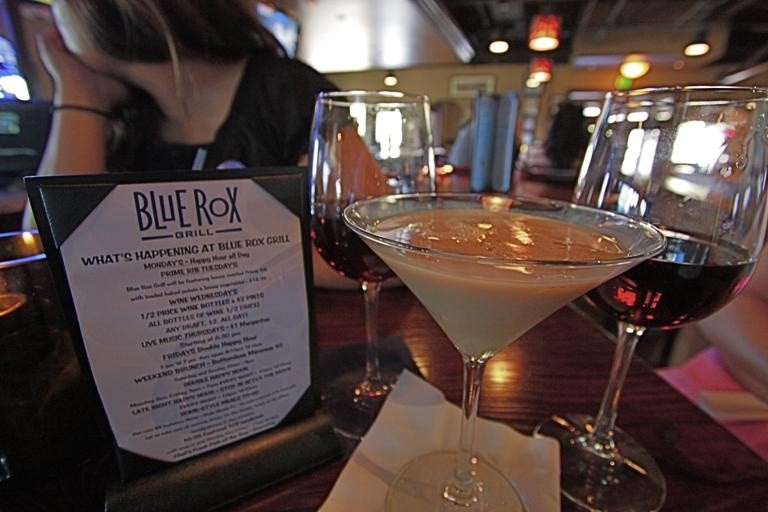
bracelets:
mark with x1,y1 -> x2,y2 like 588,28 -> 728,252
47,103 -> 110,120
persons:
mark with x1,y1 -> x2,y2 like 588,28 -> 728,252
22,2 -> 404,290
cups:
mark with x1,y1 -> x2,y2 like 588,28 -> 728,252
0,230 -> 72,426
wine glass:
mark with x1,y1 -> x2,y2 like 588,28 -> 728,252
345,193 -> 669,512
533,83 -> 768,512
308,90 -> 438,441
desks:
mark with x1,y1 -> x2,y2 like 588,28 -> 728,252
0,291 -> 768,511
